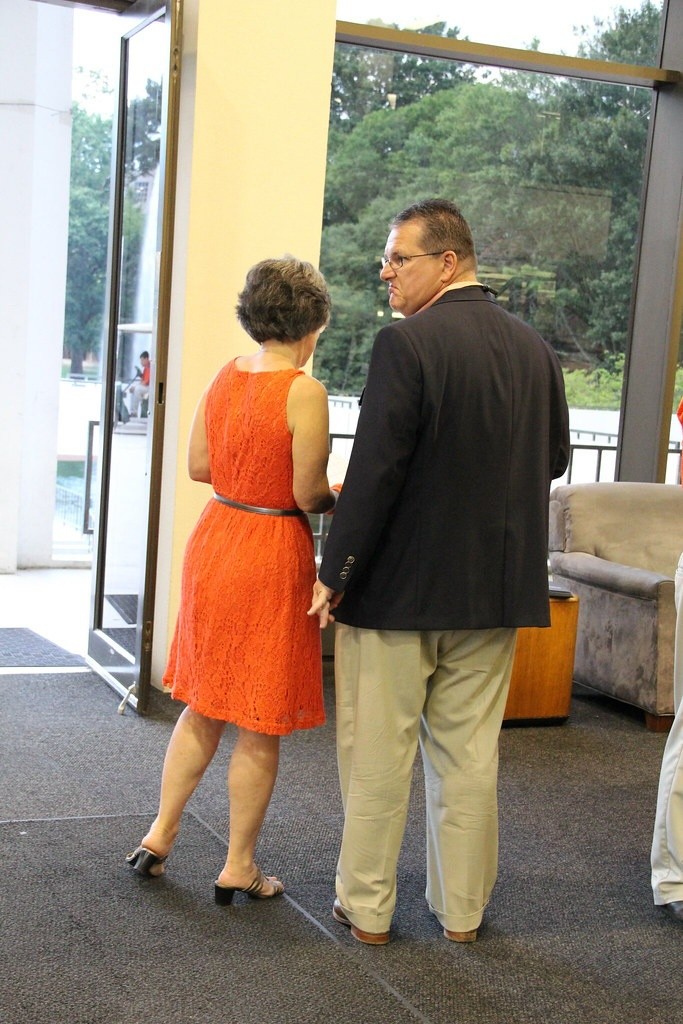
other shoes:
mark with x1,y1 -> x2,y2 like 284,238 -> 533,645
657,901 -> 683,922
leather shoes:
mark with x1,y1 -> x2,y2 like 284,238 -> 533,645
332,897 -> 390,944
442,920 -> 477,941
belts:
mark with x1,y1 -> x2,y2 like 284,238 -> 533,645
214,493 -> 303,517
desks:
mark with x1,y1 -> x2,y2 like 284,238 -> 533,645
501,593 -> 579,729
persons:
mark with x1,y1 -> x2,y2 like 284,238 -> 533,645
124,256 -> 343,908
305,199 -> 571,946
128,351 -> 151,417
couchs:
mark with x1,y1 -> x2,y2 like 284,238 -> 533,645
548,481 -> 683,732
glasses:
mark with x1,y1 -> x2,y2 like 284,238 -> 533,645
381,249 -> 457,270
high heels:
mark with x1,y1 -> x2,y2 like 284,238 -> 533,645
125,846 -> 166,877
213,870 -> 284,905
